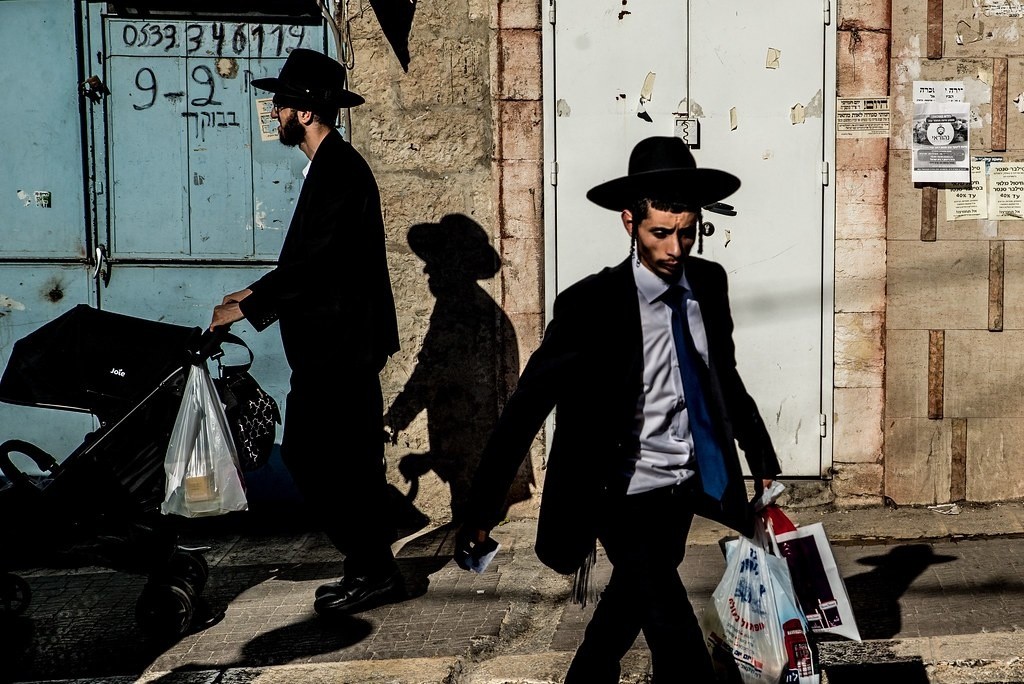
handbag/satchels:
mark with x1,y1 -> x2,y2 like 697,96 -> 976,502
212,340 -> 280,474
161,363 -> 249,519
700,481 -> 862,684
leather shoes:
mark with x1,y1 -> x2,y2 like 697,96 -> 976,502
314,566 -> 405,617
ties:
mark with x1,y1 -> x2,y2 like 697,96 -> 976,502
660,285 -> 730,502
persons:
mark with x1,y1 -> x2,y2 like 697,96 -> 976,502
210,48 -> 405,618
451,137 -> 781,684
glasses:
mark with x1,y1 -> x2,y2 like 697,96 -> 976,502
271,96 -> 299,112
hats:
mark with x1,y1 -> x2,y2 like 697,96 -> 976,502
587,136 -> 741,212
250,49 -> 367,109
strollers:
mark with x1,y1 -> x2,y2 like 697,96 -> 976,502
0,300 -> 234,645
